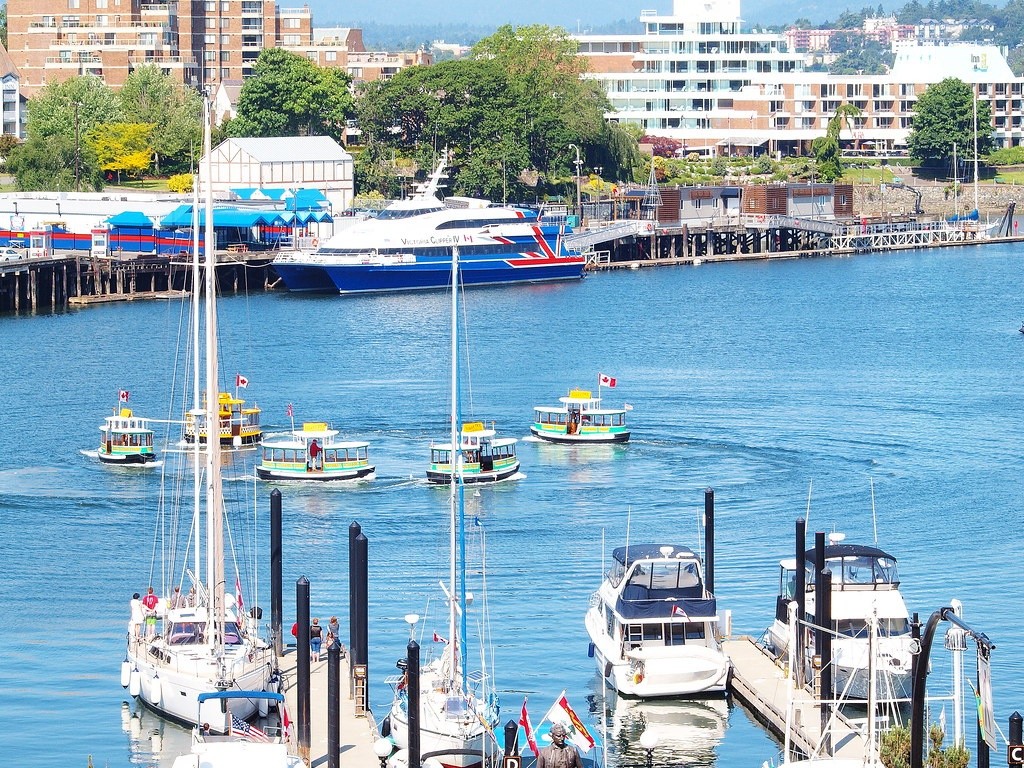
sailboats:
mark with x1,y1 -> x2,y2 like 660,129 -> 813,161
382,247 -> 502,768
123,47 -> 275,737
946,86 -> 1000,233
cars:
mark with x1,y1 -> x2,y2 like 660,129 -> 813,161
340,208 -> 383,218
0,249 -> 23,262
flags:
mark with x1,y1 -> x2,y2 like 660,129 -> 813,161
236,374 -> 250,389
433,631 -> 449,645
598,372 -> 617,388
231,714 -> 270,743
118,389 -> 129,403
518,689 -> 597,756
235,575 -> 244,628
286,403 -> 294,417
280,700 -> 291,740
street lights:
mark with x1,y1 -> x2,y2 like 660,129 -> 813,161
68,102 -> 83,193
808,160 -> 817,220
569,144 -> 582,206
862,160 -> 869,212
594,167 -> 603,230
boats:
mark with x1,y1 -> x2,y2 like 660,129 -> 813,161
766,476 -> 932,712
309,115 -> 585,295
270,166 -> 368,292
584,499 -> 733,699
253,423 -> 375,481
165,489 -> 518,768
183,392 -> 261,445
529,387 -> 631,443
97,407 -> 156,464
426,419 -> 520,485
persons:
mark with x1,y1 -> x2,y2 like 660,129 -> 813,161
465,436 -> 476,463
292,615 -> 339,663
535,722 -> 586,768
309,439 -> 323,471
221,405 -> 232,427
204,723 -> 211,736
130,586 -> 159,643
170,586 -> 197,610
325,454 -> 335,462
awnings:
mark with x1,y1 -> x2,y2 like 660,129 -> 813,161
229,188 -> 332,211
714,137 -> 770,147
